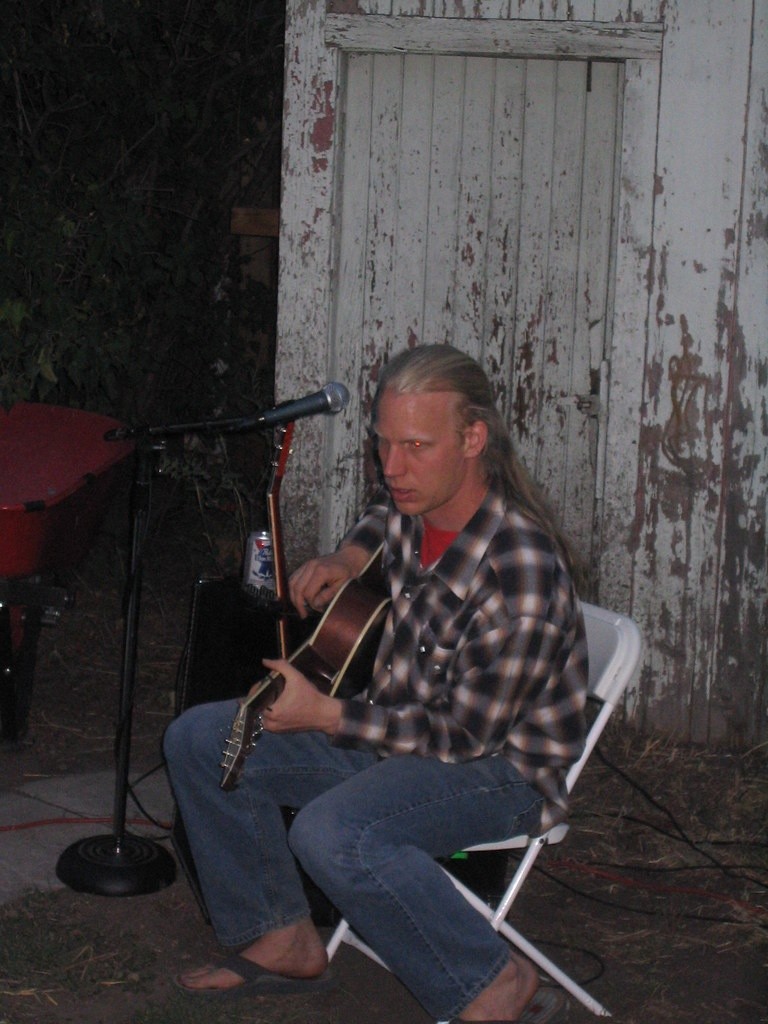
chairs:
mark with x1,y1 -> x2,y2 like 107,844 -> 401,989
327,599 -> 643,1024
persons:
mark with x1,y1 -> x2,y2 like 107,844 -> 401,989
163,345 -> 588,1024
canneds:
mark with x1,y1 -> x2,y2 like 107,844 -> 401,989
243,531 -> 273,585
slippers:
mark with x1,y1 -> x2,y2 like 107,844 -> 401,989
438,986 -> 572,1024
171,953 -> 339,1000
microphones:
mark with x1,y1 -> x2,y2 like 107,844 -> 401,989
242,381 -> 350,428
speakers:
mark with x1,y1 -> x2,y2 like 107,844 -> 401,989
178,580 -> 325,715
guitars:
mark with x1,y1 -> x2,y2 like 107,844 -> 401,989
216,536 -> 393,795
